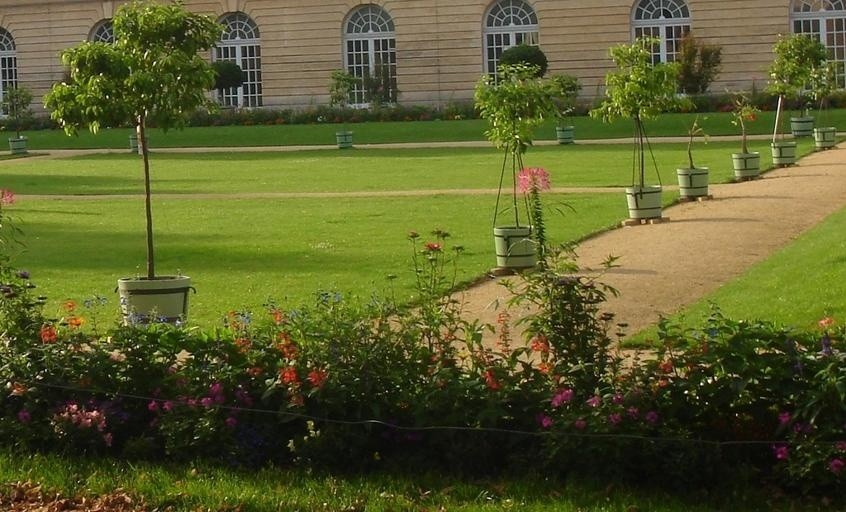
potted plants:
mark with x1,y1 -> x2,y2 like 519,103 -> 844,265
42,0 -> 231,326
328,69 -> 364,148
474,61 -> 583,267
0,82 -> 35,154
